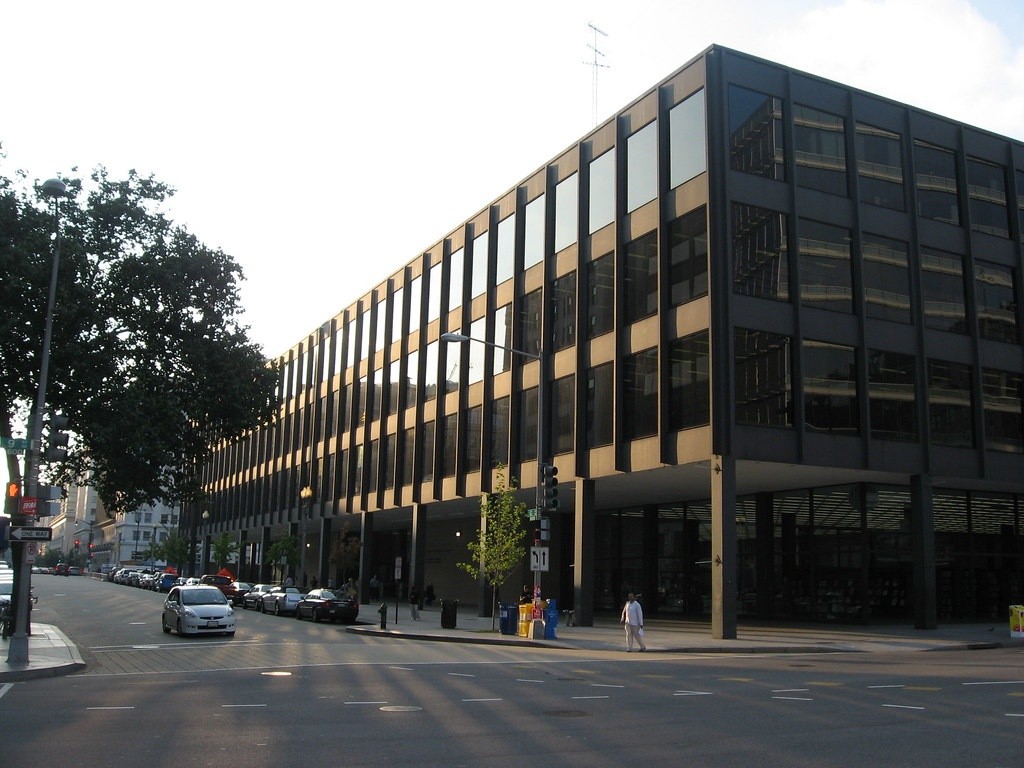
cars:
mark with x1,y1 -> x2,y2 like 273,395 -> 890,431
0,560 -> 82,604
161,585 -> 235,638
107,566 -> 201,592
295,588 -> 360,624
242,584 -> 278,612
224,581 -> 256,607
261,584 -> 310,616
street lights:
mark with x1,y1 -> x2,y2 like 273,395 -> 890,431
439,332 -> 551,621
301,486 -> 313,586
150,522 -> 159,572
200,510 -> 209,576
134,518 -> 141,564
74,519 -> 94,565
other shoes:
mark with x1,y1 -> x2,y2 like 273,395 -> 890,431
627,648 -> 632,651
638,646 -> 646,652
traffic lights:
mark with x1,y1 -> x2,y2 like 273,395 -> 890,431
89,544 -> 93,549
543,465 -> 558,509
6,482 -> 21,500
39,484 -> 63,517
75,540 -> 78,549
46,413 -> 69,462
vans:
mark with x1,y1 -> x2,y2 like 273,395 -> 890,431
194,575 -> 231,594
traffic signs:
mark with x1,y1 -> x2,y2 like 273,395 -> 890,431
8,526 -> 53,541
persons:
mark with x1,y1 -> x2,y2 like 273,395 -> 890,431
370,575 -> 380,601
144,569 -> 149,573
346,578 -> 356,591
310,576 -> 319,590
409,586 -> 420,620
620,591 -> 646,652
520,585 -> 534,604
426,582 -> 434,606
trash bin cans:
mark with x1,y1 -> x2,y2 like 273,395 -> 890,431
498,602 -> 518,635
439,598 -> 460,629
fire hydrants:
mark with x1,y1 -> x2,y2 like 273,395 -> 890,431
378,603 -> 388,629
